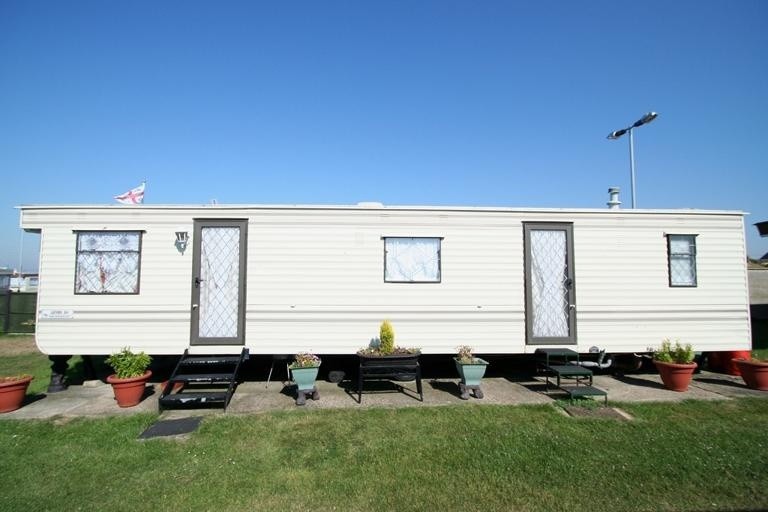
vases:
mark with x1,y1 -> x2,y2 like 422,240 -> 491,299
729,354 -> 767,392
286,364 -> 321,390
450,356 -> 490,386
0,375 -> 36,412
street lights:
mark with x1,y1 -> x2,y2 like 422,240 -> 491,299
604,109 -> 659,211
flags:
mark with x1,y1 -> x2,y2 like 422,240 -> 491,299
114,183 -> 144,204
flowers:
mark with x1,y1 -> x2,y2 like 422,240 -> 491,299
453,345 -> 474,364
291,351 -> 317,367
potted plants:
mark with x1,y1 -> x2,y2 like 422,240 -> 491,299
357,320 -> 421,372
645,338 -> 697,392
102,345 -> 155,407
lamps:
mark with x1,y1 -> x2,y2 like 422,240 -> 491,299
173,226 -> 188,249
